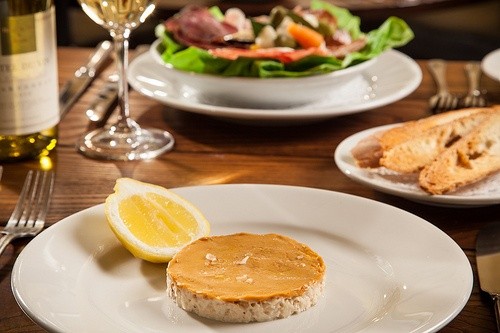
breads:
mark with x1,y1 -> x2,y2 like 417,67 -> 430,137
166,232 -> 327,323
351,105 -> 500,195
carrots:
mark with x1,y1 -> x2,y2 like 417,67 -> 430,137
288,23 -> 323,49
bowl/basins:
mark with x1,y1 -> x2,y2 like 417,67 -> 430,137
146,28 -> 381,107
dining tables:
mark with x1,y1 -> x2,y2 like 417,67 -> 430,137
0,46 -> 500,333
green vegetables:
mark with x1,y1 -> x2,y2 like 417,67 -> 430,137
159,0 -> 414,78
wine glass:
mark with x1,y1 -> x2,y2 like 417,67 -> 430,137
75,0 -> 177,161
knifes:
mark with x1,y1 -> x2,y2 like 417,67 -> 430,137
472,223 -> 500,332
60,41 -> 113,119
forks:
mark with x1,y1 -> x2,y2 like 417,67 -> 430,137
461,62 -> 487,107
0,169 -> 54,256
427,59 -> 458,110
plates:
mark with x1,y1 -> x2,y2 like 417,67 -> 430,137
333,123 -> 500,207
128,44 -> 422,119
482,48 -> 500,86
11,184 -> 473,332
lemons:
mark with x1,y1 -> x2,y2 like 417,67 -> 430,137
104,178 -> 211,263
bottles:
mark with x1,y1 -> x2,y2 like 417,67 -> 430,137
0,1 -> 60,165
